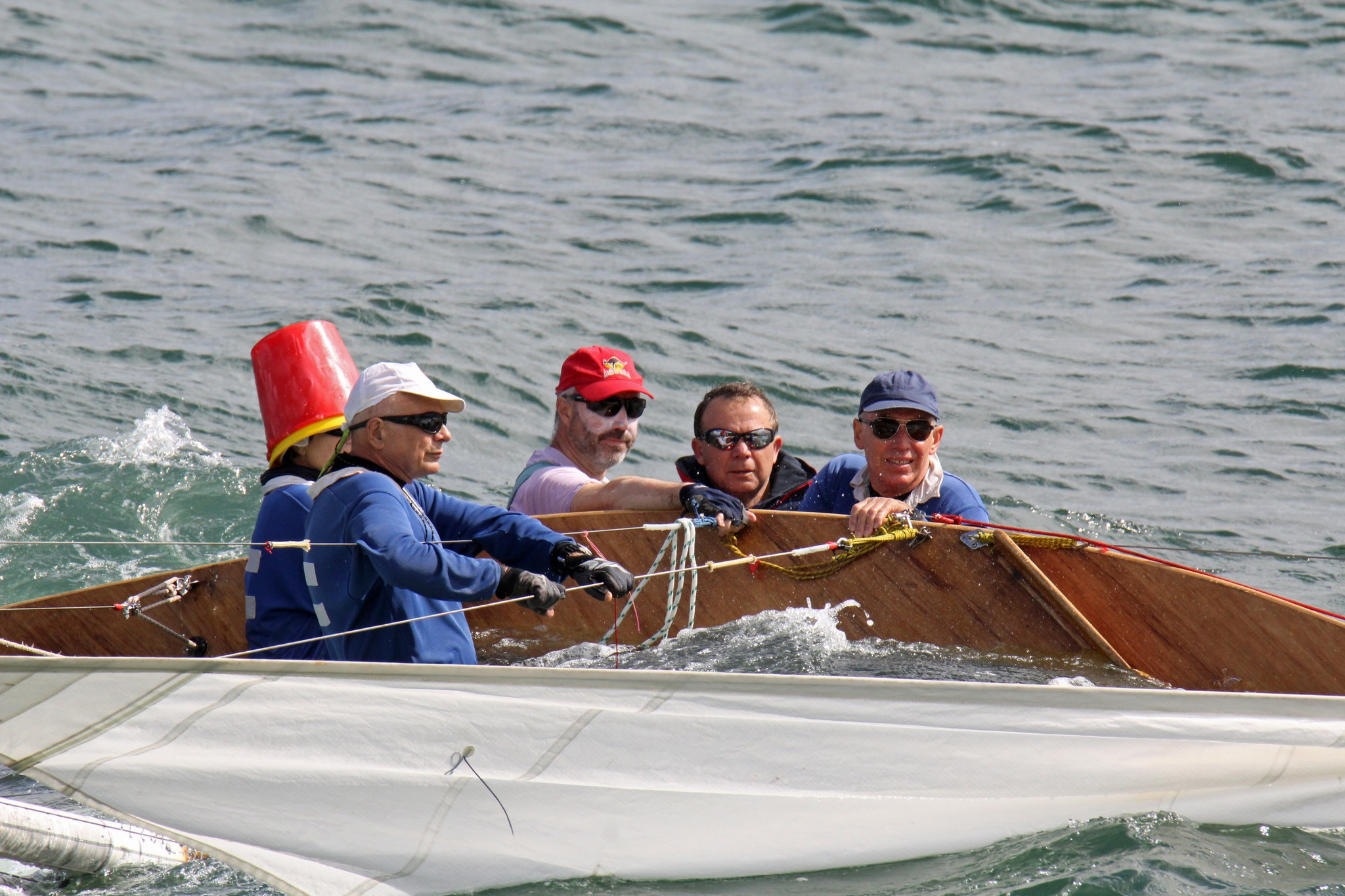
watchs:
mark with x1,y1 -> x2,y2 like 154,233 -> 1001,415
556,543 -> 592,569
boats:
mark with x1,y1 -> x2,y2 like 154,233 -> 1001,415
0,504 -> 1343,896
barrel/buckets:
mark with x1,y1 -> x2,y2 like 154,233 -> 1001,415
251,319 -> 361,468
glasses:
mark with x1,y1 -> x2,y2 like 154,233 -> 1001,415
378,412 -> 448,433
327,428 -> 351,437
698,429 -> 775,450
562,394 -> 646,418
858,417 -> 937,441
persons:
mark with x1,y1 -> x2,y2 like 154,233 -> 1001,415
302,362 -> 634,664
674,382 -> 817,513
798,371 -> 990,539
245,320 -> 362,661
506,345 -> 756,536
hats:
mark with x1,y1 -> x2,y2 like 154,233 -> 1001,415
556,345 -> 654,401
858,370 -> 940,420
344,362 -> 466,425
